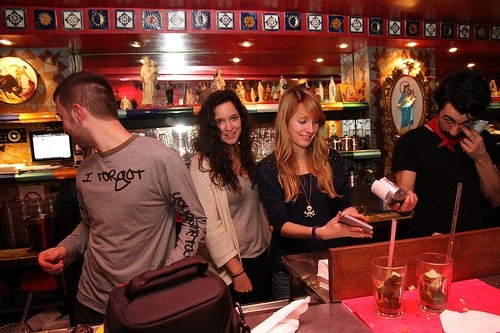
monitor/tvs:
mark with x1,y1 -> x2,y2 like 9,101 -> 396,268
28,130 -> 73,166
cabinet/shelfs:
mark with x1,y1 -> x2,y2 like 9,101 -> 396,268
0,98 -> 500,265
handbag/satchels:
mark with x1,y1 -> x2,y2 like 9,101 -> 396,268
104,255 -> 251,333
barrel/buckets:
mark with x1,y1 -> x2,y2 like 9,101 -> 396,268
21,213 -> 58,250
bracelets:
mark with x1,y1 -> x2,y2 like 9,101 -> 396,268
233,270 -> 245,278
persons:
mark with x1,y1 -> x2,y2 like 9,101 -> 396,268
38,72 -> 207,328
189,90 -> 272,305
257,87 -> 373,300
235,81 -> 246,101
397,85 -> 416,127
388,72 -> 500,236
140,56 -> 159,104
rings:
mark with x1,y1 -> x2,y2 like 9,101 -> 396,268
338,211 -> 344,221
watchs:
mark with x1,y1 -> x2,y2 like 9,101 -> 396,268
312,226 -> 320,240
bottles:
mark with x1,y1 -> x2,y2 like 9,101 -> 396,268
366,169 -> 377,185
74,145 -> 84,166
347,167 -> 358,188
325,131 -> 370,151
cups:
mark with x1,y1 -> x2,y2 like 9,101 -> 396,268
415,253 -> 454,315
371,256 -> 408,319
144,126 -> 198,165
0,323 -> 31,333
0,192 -> 61,248
248,126 -> 275,158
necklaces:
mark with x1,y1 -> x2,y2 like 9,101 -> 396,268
299,172 -> 315,217
